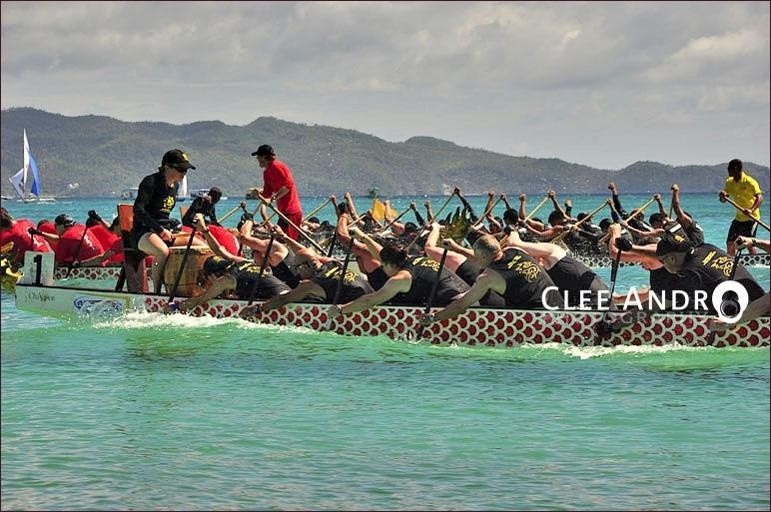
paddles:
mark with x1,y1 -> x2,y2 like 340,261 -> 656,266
319,215 -> 366,247
551,200 -> 608,242
499,196 -> 550,250
597,198 -> 655,246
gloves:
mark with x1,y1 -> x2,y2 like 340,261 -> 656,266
27,226 -> 43,236
613,237 -> 633,253
86,210 -> 103,221
592,321 -> 612,338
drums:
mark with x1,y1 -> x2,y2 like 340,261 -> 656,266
162,244 -> 212,295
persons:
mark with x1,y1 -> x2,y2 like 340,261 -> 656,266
0,145 -> 771,327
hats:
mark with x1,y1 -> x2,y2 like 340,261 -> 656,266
204,255 -> 235,272
250,146 -> 277,158
55,214 -> 75,227
162,148 -> 196,173
656,233 -> 691,257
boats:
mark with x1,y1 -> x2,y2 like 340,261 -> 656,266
729,252 -> 771,271
565,253 -> 645,272
11,276 -> 770,350
0,267 -> 131,287
